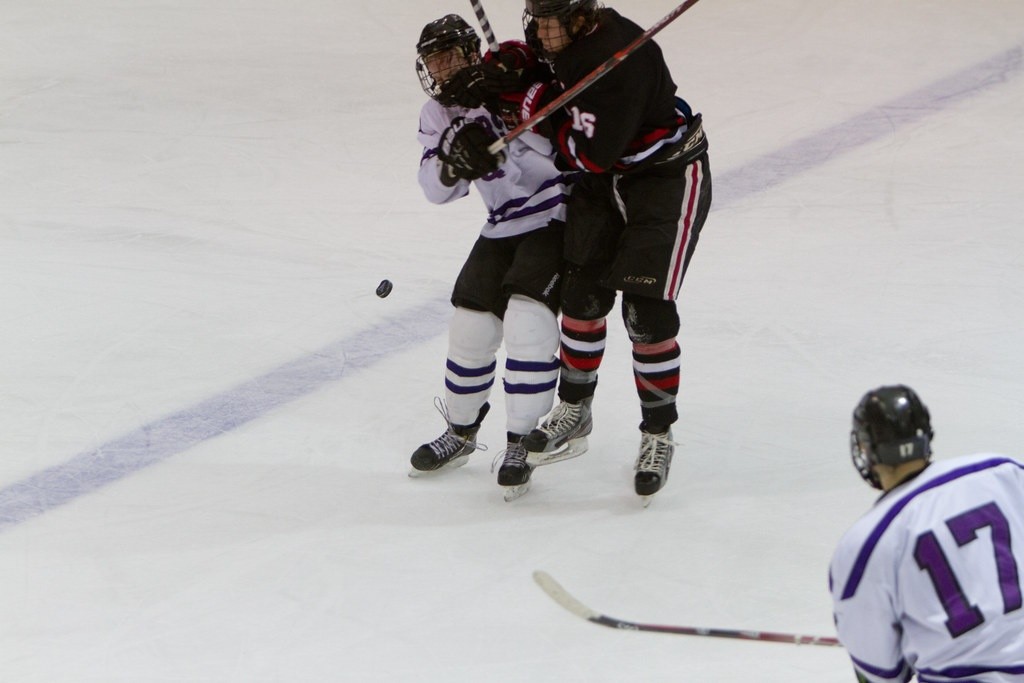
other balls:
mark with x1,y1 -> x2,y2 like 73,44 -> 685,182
375,278 -> 395,299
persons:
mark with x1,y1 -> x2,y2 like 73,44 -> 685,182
829,385 -> 1024,683
408,0 -> 712,508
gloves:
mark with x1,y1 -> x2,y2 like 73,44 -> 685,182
437,116 -> 506,177
497,82 -> 548,133
482,39 -> 535,93
440,64 -> 486,108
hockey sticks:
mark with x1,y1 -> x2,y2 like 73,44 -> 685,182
486,0 -> 701,159
529,565 -> 848,650
466,0 -> 505,61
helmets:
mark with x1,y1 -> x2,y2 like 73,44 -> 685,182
851,383 -> 934,490
416,14 -> 481,104
525,0 -> 599,61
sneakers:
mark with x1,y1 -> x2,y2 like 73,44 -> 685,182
491,431 -> 537,502
522,373 -> 598,466
408,396 -> 491,478
633,421 -> 685,508
